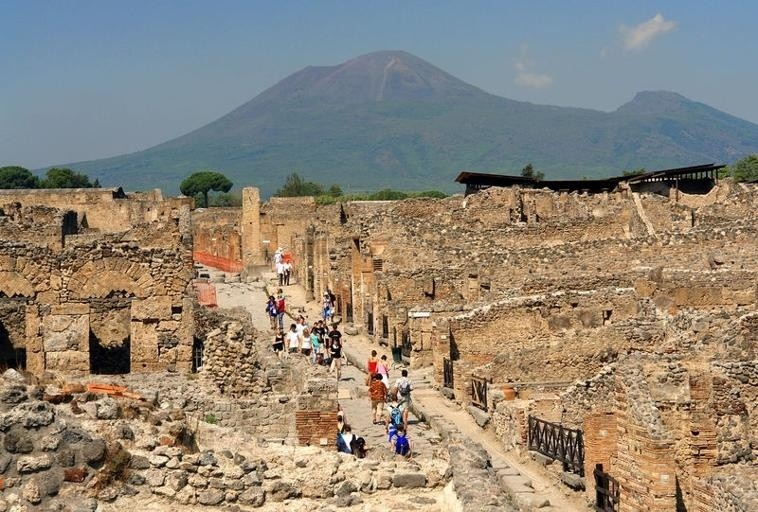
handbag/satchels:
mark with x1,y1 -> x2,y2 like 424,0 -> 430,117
349,434 -> 356,455
398,388 -> 408,396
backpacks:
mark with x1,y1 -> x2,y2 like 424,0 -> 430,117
394,433 -> 409,456
389,404 -> 402,425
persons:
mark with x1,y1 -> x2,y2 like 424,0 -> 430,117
263,246 -> 417,459
